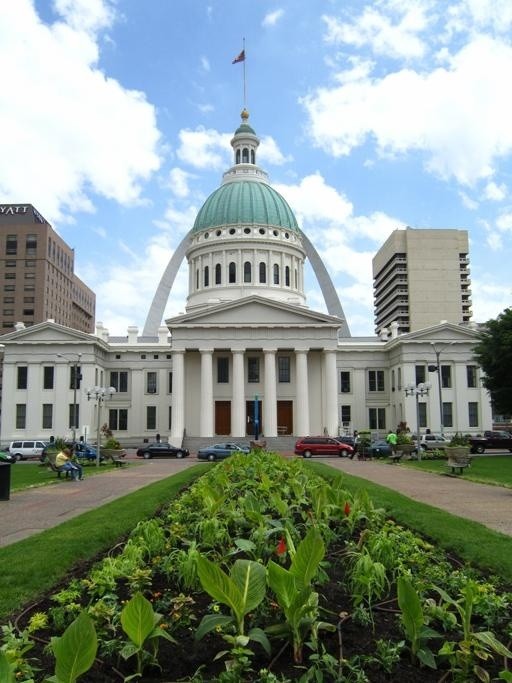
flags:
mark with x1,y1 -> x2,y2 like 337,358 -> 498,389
231,48 -> 245,64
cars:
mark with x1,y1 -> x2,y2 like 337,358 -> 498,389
333,436 -> 363,454
137,442 -> 190,459
364,438 -> 396,458
41,442 -> 104,465
198,442 -> 251,461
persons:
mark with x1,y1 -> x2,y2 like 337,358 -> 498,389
350,431 -> 361,459
56,448 -> 79,481
69,449 -> 85,480
387,430 -> 397,453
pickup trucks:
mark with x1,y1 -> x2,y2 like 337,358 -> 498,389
468,431 -> 512,454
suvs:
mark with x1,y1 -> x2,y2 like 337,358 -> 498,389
419,434 -> 452,452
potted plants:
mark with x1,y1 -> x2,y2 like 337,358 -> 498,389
100,439 -> 124,463
48,439 -> 69,465
444,432 -> 472,463
397,432 -> 417,459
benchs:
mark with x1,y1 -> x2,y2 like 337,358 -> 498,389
111,455 -> 126,467
445,455 -> 472,474
388,450 -> 404,463
49,460 -> 69,478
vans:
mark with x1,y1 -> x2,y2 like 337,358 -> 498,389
295,436 -> 357,459
8,440 -> 48,461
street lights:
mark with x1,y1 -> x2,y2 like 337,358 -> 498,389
429,339 -> 456,444
86,385 -> 116,466
56,351 -> 82,443
